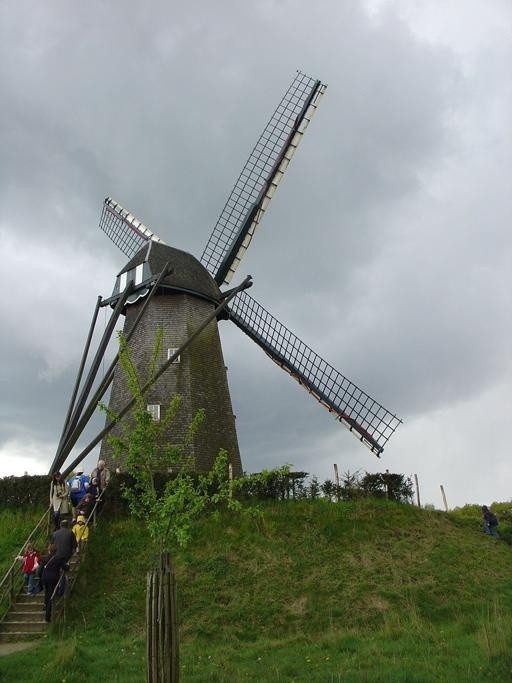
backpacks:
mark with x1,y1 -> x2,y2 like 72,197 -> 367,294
70,475 -> 84,492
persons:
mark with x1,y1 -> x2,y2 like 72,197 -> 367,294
65,471 -> 89,521
49,518 -> 78,565
480,503 -> 499,535
89,458 -> 111,510
73,476 -> 100,522
15,544 -> 41,594
37,541 -> 71,622
72,515 -> 89,543
90,476 -> 101,498
47,471 -> 69,519
